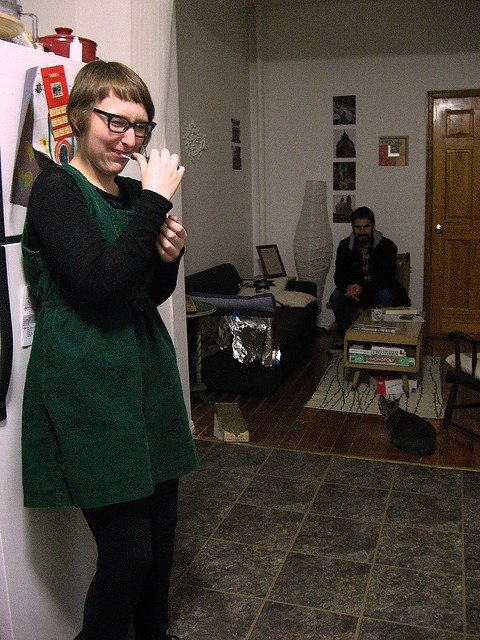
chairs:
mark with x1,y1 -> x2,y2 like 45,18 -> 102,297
326,252 -> 411,326
441,331 -> 480,441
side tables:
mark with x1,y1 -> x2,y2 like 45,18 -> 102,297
186,297 -> 217,407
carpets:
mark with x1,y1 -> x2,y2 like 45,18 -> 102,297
304,350 -> 446,419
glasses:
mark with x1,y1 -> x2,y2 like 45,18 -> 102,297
94,108 -> 157,138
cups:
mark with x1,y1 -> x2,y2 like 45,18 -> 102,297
371,305 -> 383,324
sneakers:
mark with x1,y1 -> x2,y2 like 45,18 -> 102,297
328,339 -> 344,353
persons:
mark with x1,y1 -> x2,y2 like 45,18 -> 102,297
22,62 -> 202,640
325,205 -> 412,356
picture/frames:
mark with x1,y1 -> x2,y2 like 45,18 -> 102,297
256,245 -> 286,278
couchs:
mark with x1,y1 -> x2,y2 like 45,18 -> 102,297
185,263 -> 318,397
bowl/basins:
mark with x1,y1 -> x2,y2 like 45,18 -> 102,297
0,12 -> 24,40
